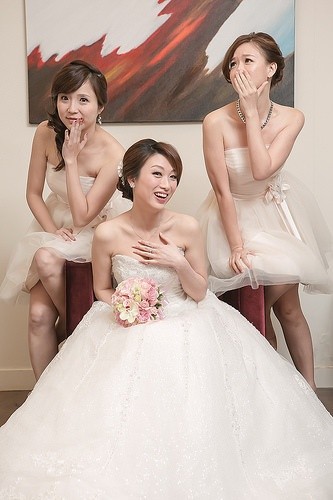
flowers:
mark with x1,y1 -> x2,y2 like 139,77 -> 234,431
109,273 -> 171,329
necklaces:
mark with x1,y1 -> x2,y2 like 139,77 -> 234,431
131,210 -> 166,241
235,100 -> 273,132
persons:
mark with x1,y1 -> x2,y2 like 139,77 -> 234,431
0,61 -> 125,382
203,32 -> 333,393
0,139 -> 333,500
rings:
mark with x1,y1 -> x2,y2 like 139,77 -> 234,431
148,248 -> 153,252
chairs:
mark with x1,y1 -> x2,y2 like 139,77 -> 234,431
65,259 -> 266,338
